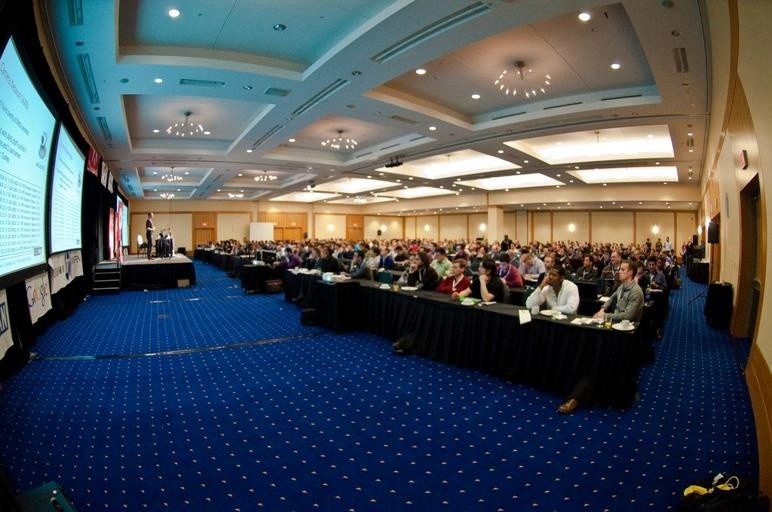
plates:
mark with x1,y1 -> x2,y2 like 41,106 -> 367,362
613,321 -> 635,332
380,286 -> 391,289
461,300 -> 475,306
401,285 -> 419,292
540,309 -> 562,316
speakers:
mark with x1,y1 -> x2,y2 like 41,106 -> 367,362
703,281 -> 733,330
178,248 -> 186,256
708,222 -> 719,244
693,235 -> 698,246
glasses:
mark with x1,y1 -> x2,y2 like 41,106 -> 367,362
544,261 -> 553,263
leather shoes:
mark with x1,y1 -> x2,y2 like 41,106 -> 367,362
558,398 -> 580,413
393,341 -> 404,353
657,328 -> 662,340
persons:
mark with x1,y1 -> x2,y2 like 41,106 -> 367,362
416,236 -> 545,302
682,237 -> 693,256
201,239 -> 421,284
392,252 -> 438,352
558,261 -> 644,411
525,236 -> 679,338
146,211 -> 155,260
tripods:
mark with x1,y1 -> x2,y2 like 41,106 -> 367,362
688,245 -> 721,305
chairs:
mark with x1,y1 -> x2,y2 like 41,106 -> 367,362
195,238 -> 678,412
136,234 -> 148,258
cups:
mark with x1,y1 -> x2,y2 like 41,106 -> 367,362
603,313 -> 613,330
464,297 -> 472,303
621,320 -> 630,329
531,305 -> 540,315
393,281 -> 400,292
382,284 -> 388,288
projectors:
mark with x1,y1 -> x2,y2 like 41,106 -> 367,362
385,162 -> 402,168
